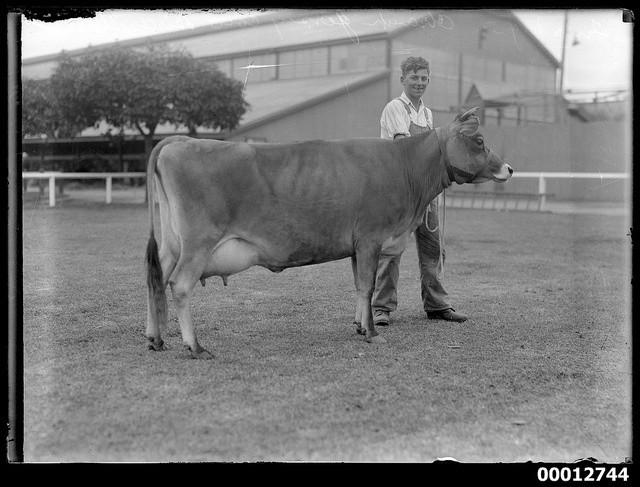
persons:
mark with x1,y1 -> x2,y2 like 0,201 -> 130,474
371,55 -> 469,324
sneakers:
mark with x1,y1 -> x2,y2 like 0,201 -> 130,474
427,308 -> 469,322
373,310 -> 390,325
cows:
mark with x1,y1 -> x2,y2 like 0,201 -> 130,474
143,106 -> 514,360
